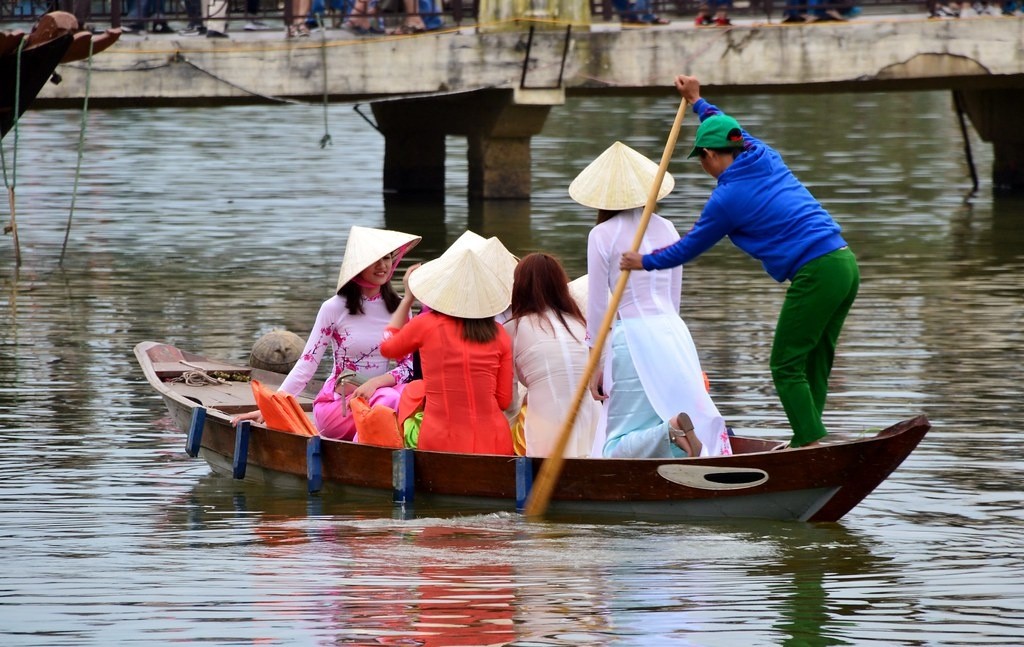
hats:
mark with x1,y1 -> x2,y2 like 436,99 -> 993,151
686,114 -> 744,160
567,273 -> 619,324
567,140 -> 675,210
441,230 -> 521,264
408,249 -> 512,320
473,237 -> 519,297
335,225 -> 422,294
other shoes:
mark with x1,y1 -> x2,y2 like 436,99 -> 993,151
346,20 -> 383,34
621,18 -> 654,29
206,30 -> 227,37
121,25 -> 145,35
245,23 -> 269,29
651,17 -> 670,25
178,26 -> 202,36
288,23 -> 309,38
390,25 -> 424,34
151,22 -> 175,34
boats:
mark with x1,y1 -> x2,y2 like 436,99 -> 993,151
0,11 -> 125,145
134,341 -> 933,525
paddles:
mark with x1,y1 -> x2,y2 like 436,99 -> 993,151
524,96 -> 689,518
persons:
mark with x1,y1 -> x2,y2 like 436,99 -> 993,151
695,0 -> 732,26
290,0 -> 443,38
568,141 -> 732,458
231,226 -> 421,441
613,0 -> 670,25
224,0 -> 268,30
179,0 -> 203,36
119,0 -> 175,35
379,249 -> 514,455
503,254 -> 603,458
443,232 -> 527,429
620,77 -> 860,448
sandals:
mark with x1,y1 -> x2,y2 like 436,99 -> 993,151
668,412 -> 702,457
